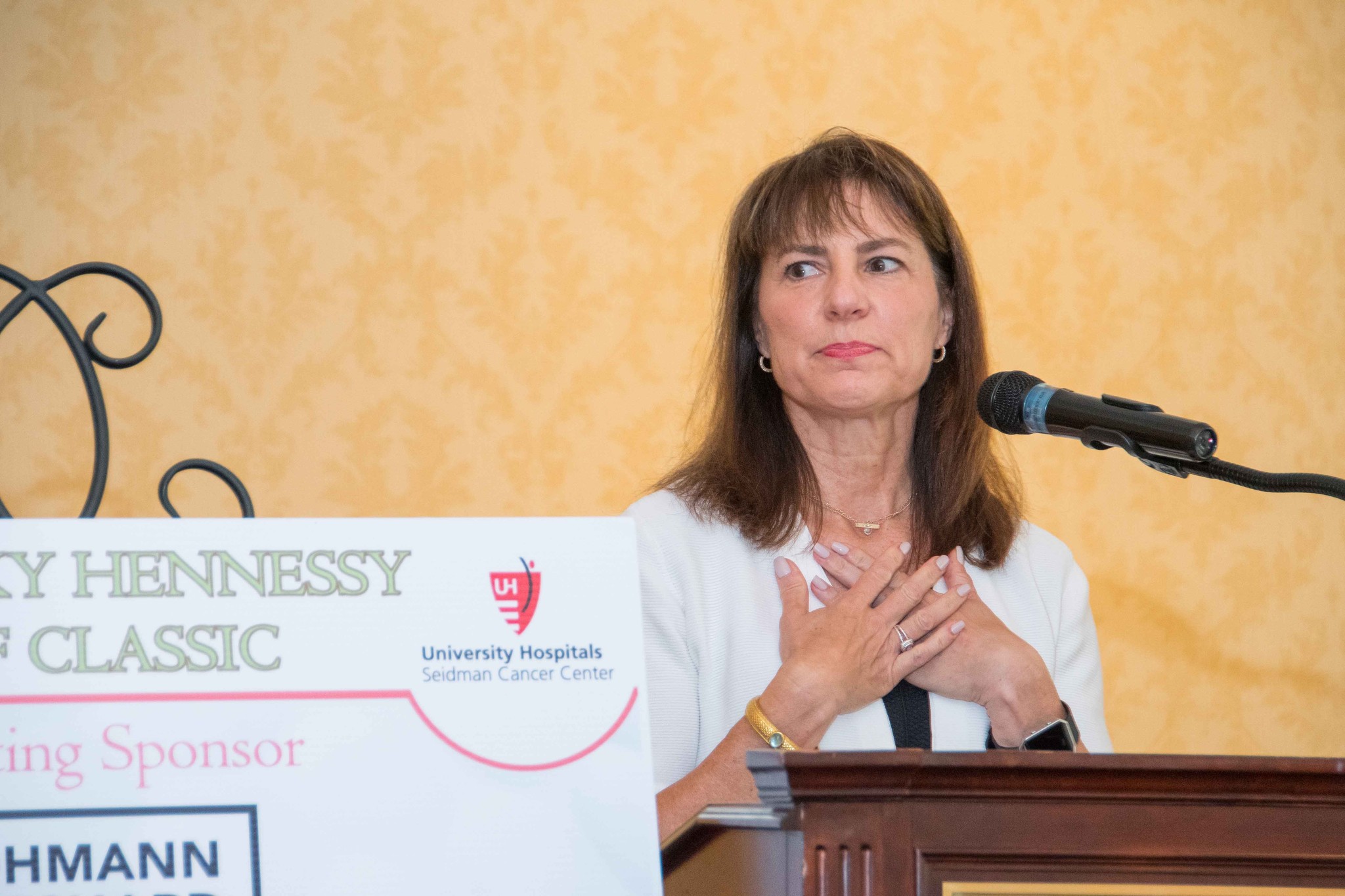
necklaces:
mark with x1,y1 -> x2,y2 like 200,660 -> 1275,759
801,490 -> 915,537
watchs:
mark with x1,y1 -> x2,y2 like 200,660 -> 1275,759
985,700 -> 1080,752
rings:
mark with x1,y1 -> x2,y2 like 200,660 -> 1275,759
893,625 -> 914,655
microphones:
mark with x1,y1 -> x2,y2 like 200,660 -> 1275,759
976,370 -> 1218,463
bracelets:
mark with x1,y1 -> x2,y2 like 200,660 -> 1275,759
744,695 -> 802,751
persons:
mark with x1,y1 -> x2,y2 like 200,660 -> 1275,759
629,132 -> 1116,853
